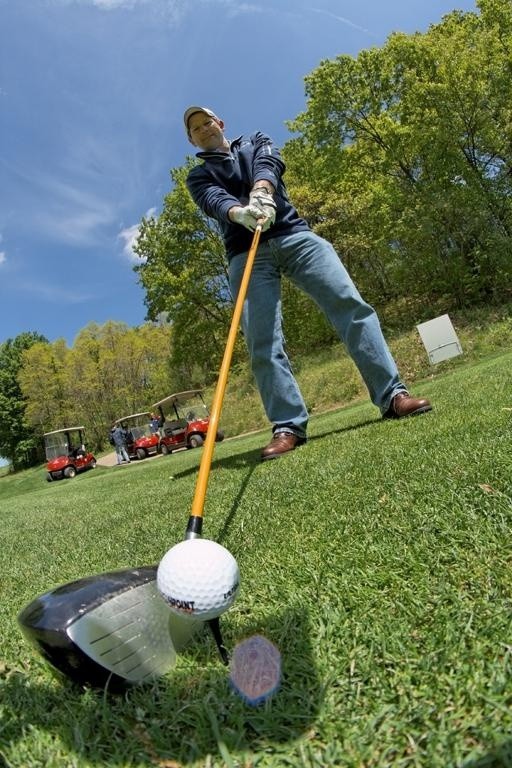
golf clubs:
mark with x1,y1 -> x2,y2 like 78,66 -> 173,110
18,219 -> 261,698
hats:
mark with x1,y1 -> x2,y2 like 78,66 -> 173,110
183,106 -> 220,128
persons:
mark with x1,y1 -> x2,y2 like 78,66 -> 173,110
150,413 -> 162,440
109,422 -> 130,465
183,106 -> 432,460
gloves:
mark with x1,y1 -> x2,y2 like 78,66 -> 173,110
233,186 -> 278,233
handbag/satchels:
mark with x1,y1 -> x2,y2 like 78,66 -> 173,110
110,438 -> 115,445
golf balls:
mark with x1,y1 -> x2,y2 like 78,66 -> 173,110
158,536 -> 237,621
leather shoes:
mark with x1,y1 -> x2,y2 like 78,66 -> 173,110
260,427 -> 307,461
381,391 -> 433,419
